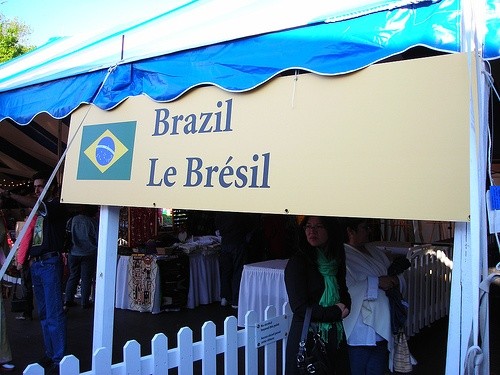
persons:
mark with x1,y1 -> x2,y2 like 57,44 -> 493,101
341,218 -> 399,375
62,205 -> 97,309
0,170 -> 67,375
284,216 -> 351,375
15,212 -> 36,321
218,210 -> 295,310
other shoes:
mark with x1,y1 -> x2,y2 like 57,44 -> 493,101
221,298 -> 228,305
0,362 -> 15,372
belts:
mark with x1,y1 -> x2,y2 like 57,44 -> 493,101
33,252 -> 59,262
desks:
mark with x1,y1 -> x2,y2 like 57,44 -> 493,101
238,259 -> 291,327
115,254 -> 224,314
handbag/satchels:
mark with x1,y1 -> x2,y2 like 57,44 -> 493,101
296,306 -> 330,375
63,217 -> 73,249
10,267 -> 34,312
392,331 -> 413,373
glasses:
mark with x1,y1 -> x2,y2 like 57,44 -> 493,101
303,225 -> 326,230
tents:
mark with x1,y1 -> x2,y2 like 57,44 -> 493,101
0,0 -> 500,375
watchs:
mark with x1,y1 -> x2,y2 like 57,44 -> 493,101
5,189 -> 11,198
